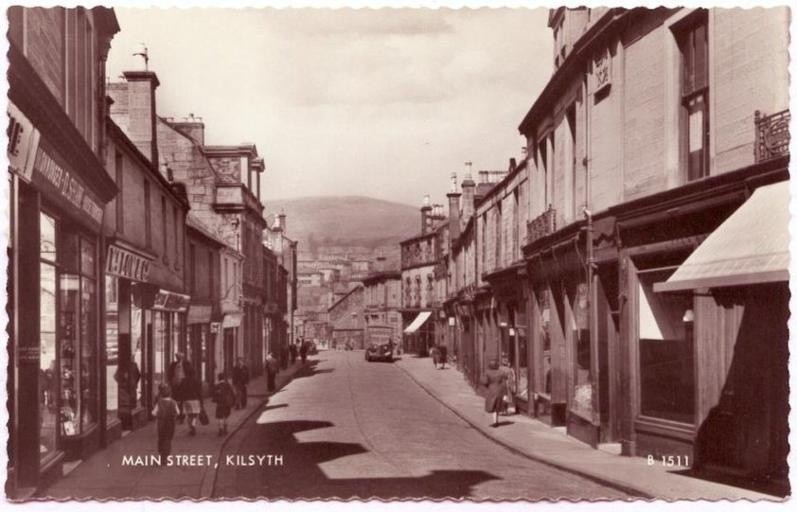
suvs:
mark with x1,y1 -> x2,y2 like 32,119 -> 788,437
364,334 -> 394,362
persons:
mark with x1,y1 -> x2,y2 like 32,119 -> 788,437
479,360 -> 508,427
150,385 -> 180,467
167,353 -> 195,400
177,365 -> 202,434
231,357 -> 249,409
299,344 -> 306,364
279,342 -> 288,370
38,360 -> 54,436
265,352 -> 279,392
393,335 -> 400,358
289,341 -> 297,364
428,343 -> 440,369
211,372 -> 235,436
